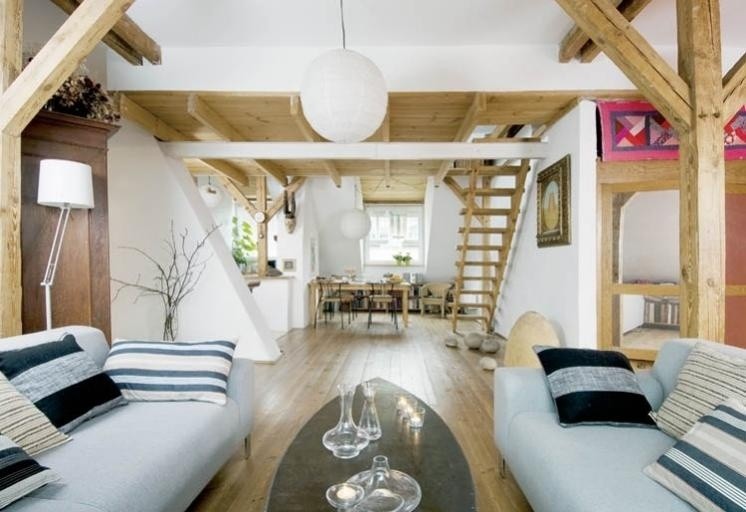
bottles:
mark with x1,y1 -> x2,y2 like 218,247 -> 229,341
343,454 -> 423,512
321,380 -> 383,460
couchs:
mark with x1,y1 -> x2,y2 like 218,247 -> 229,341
0,324 -> 257,512
493,336 -> 746,511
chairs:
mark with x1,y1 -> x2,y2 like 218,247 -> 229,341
314,276 -> 399,330
420,282 -> 453,318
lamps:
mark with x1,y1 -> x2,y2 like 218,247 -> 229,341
37,159 -> 95,330
299,0 -> 389,142
340,175 -> 370,240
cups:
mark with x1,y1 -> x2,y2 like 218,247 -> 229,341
393,391 -> 426,428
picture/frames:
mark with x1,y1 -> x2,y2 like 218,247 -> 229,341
282,258 -> 296,273
536,154 -> 571,247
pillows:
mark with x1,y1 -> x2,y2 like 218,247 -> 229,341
102,336 -> 241,405
647,340 -> 745,441
0,331 -> 130,434
642,396 -> 746,512
0,434 -> 62,511
532,343 -> 659,429
0,371 -> 74,458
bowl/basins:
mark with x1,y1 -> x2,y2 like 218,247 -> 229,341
325,483 -> 364,511
